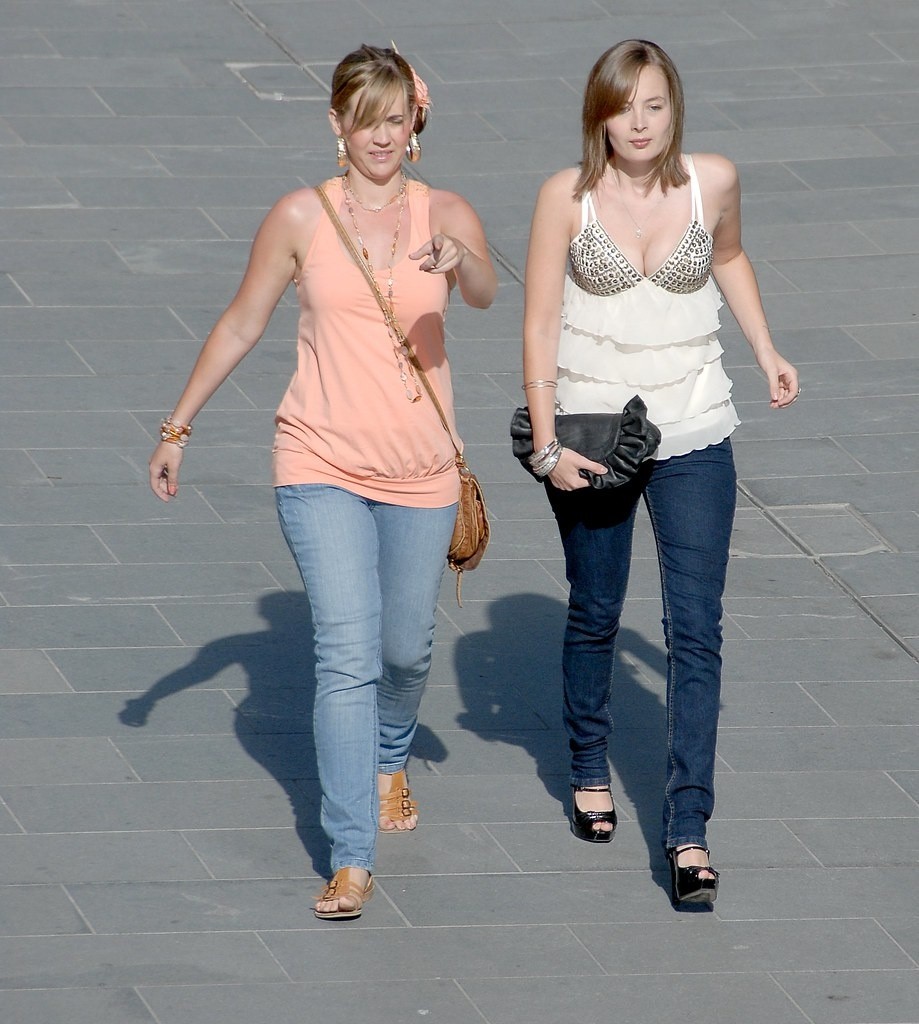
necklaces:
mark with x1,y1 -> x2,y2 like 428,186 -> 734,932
611,169 -> 663,239
342,169 -> 423,401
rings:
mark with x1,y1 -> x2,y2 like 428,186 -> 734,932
798,388 -> 801,393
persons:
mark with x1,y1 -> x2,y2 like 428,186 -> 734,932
520,40 -> 801,908
148,45 -> 499,920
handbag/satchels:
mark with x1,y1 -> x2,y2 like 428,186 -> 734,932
508,394 -> 661,492
448,472 -> 491,573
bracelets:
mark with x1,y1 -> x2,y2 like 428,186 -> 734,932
521,380 -> 558,390
160,417 -> 192,448
527,439 -> 562,477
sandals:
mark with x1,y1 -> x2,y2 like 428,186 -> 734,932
375,766 -> 418,834
310,869 -> 375,919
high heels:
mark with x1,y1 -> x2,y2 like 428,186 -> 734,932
570,781 -> 618,842
670,841 -> 719,905
